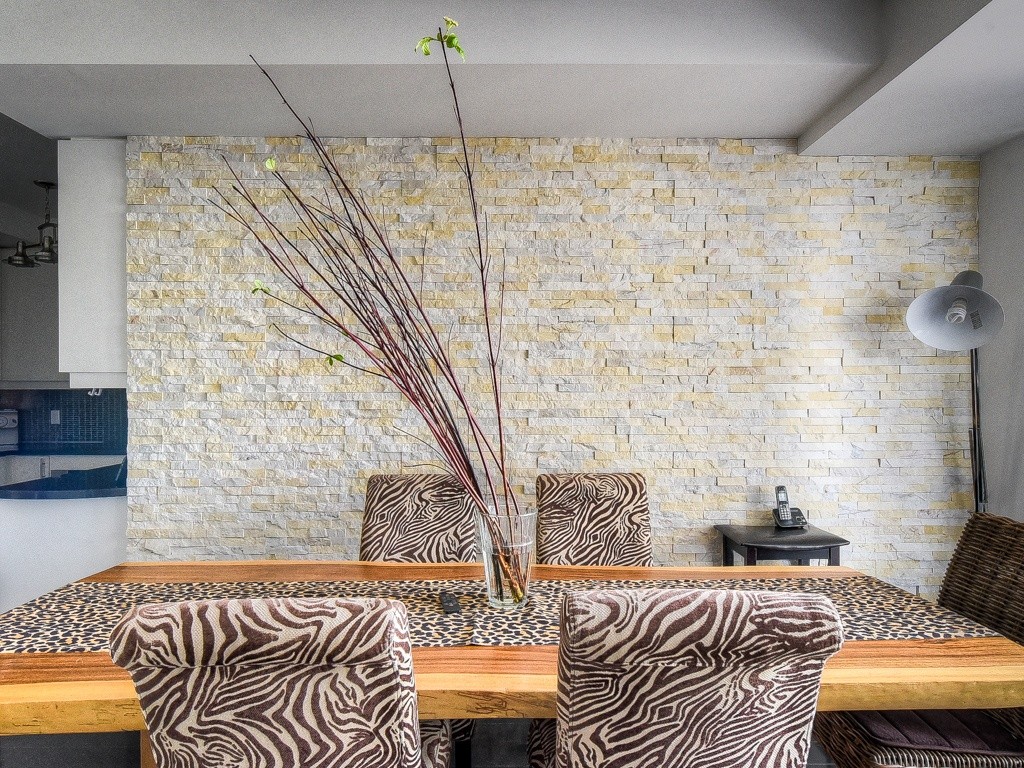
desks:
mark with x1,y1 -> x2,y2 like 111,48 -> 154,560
714,522 -> 846,570
1,558 -> 1024,741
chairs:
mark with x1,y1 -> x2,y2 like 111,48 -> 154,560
358,474 -> 478,737
535,470 -> 655,572
108,597 -> 454,768
815,515 -> 1024,768
539,586 -> 845,768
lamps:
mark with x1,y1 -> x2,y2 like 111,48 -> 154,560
7,179 -> 58,268
905,270 -> 1005,516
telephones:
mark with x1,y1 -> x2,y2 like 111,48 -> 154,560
772,485 -> 807,529
